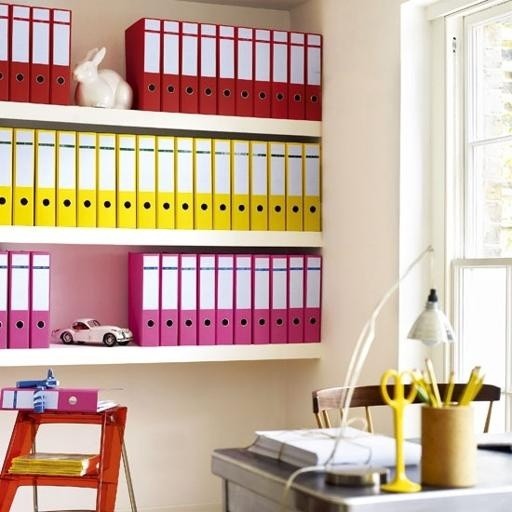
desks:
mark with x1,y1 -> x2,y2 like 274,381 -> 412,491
209,428 -> 512,510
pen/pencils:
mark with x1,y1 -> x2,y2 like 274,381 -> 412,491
412,358 -> 486,409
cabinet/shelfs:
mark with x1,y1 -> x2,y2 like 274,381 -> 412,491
0,99 -> 321,370
2,398 -> 140,512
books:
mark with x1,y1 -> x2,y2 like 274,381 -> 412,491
9,453 -> 98,477
246,427 -> 421,469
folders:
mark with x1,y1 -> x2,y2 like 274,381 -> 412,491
128,251 -> 323,346
0,3 -> 72,105
125,17 -> 322,122
0,250 -> 51,349
0,126 -> 321,231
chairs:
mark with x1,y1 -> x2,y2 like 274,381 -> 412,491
309,381 -> 502,433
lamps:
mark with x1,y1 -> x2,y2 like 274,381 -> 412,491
320,241 -> 468,489
